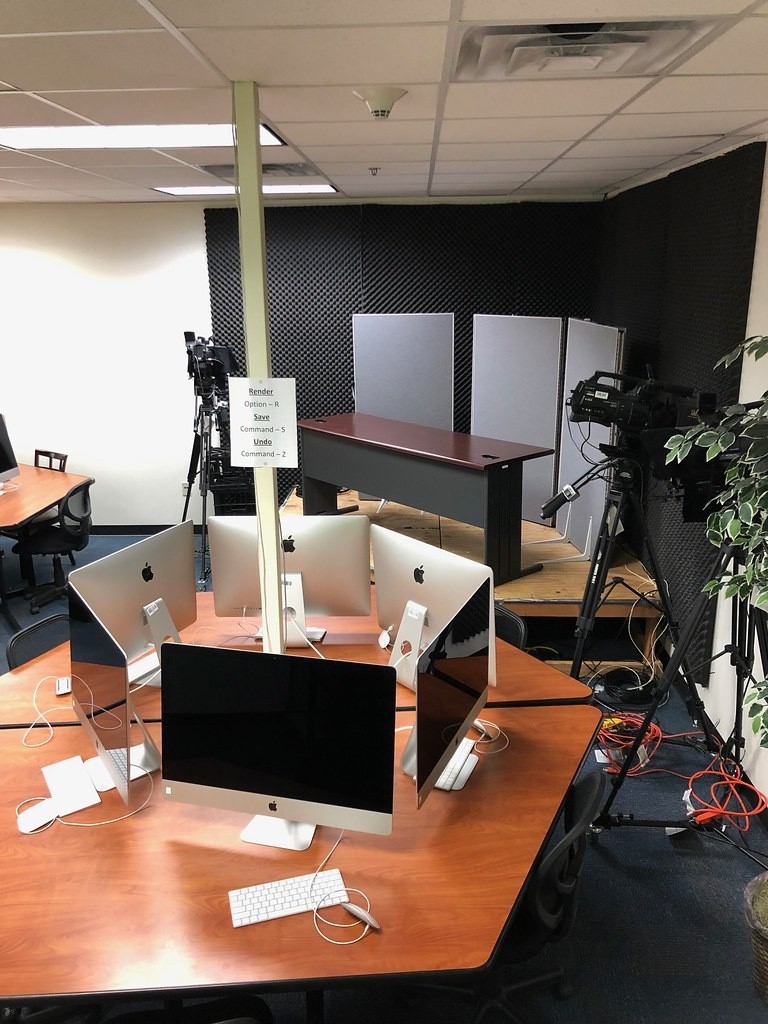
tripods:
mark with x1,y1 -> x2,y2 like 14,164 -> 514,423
540,456 -> 721,754
594,526 -> 768,870
182,393 -> 255,592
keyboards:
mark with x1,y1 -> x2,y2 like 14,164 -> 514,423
255,627 -> 327,642
106,747 -> 128,788
432,738 -> 475,791
227,868 -> 349,929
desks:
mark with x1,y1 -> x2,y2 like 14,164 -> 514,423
1,459 -> 96,598
0,585 -> 609,1002
297,411 -> 555,594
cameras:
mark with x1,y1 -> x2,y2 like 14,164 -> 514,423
567,371 -> 717,463
185,332 -> 239,396
640,401 -> 764,523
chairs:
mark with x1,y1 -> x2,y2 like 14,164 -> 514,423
6,449 -> 97,614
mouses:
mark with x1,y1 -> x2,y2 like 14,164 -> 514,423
377,629 -> 390,648
17,797 -> 58,833
340,902 -> 380,929
472,719 -> 489,736
56,677 -> 71,696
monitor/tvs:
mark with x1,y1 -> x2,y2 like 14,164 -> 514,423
369,523 -> 497,687
68,582 -> 130,810
208,516 -> 372,617
0,413 -> 21,483
413,576 -> 491,811
69,519 -> 196,665
159,643 -> 396,835
